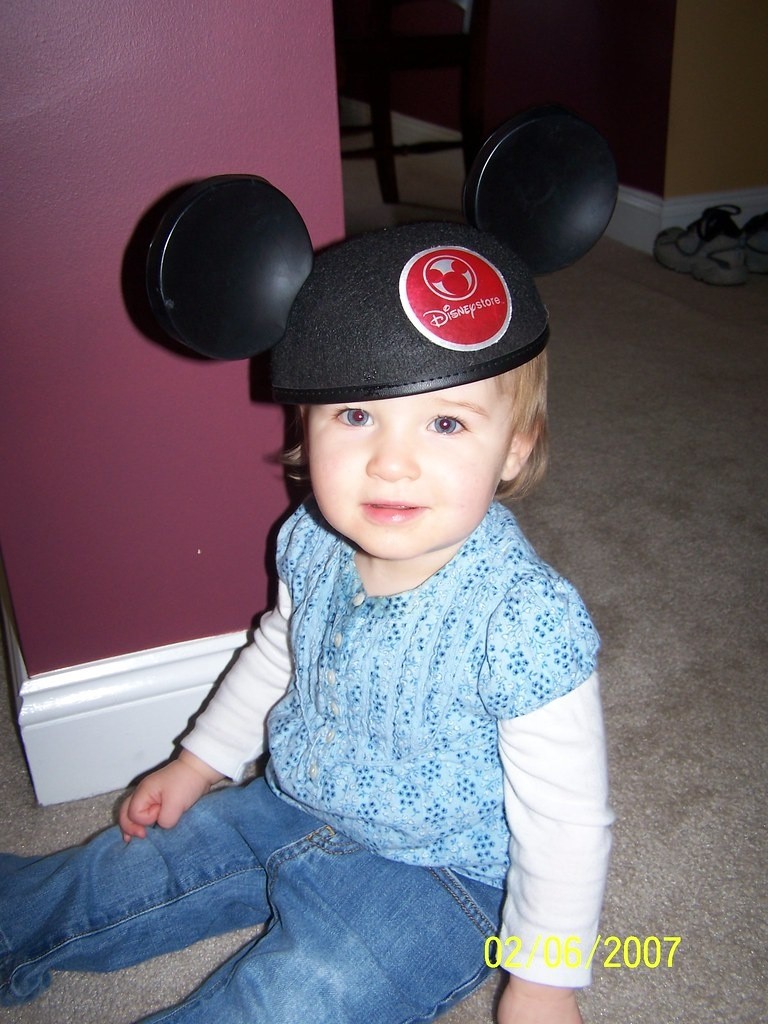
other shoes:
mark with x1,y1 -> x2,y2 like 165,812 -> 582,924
656,204 -> 768,286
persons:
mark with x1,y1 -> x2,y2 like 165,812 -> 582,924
0,219 -> 614,1024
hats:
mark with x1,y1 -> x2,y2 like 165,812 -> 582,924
146,103 -> 617,403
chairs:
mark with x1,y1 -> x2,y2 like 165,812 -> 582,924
336,0 -> 478,204
